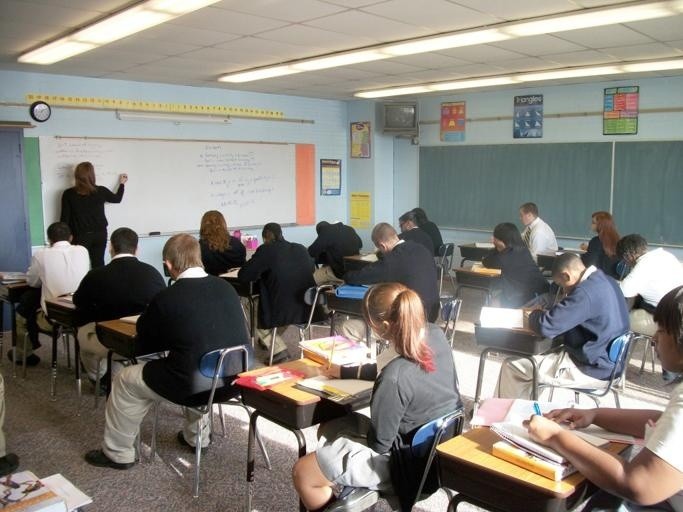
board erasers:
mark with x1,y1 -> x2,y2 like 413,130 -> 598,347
149,232 -> 160,235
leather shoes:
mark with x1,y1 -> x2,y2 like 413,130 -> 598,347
84,448 -> 134,470
7,349 -> 40,366
0,454 -> 20,475
177,429 -> 206,454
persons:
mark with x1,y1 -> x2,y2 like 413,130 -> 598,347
59,161 -> 127,271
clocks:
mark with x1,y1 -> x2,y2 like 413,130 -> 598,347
29,100 -> 52,123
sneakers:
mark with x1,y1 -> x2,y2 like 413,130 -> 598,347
263,350 -> 291,364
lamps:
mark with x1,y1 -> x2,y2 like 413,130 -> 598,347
213,0 -> 683,87
350,54 -> 682,101
14,1 -> 217,69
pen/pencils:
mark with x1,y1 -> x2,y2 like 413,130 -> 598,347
534,403 -> 541,416
328,340 -> 335,369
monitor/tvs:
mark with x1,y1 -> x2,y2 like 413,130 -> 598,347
380,102 -> 420,135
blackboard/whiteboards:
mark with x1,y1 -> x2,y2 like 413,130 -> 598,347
418,140 -> 683,248
38,135 -> 296,245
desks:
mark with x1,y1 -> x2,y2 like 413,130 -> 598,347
0,238 -> 636,512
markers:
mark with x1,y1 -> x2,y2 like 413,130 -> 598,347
123,176 -> 127,178
